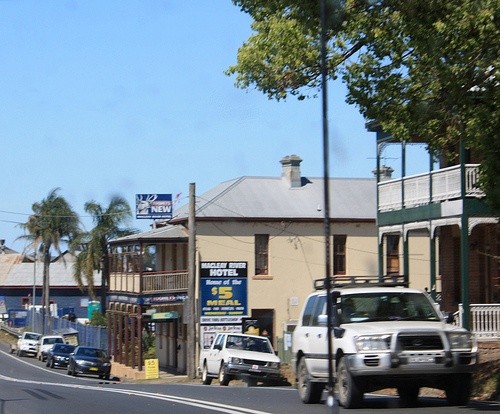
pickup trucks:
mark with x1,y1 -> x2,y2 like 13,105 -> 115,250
199,333 -> 280,388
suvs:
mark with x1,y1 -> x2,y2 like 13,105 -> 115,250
290,274 -> 480,410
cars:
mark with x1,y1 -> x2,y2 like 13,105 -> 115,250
16,332 -> 45,357
67,346 -> 112,379
45,343 -> 75,368
36,336 -> 64,362
